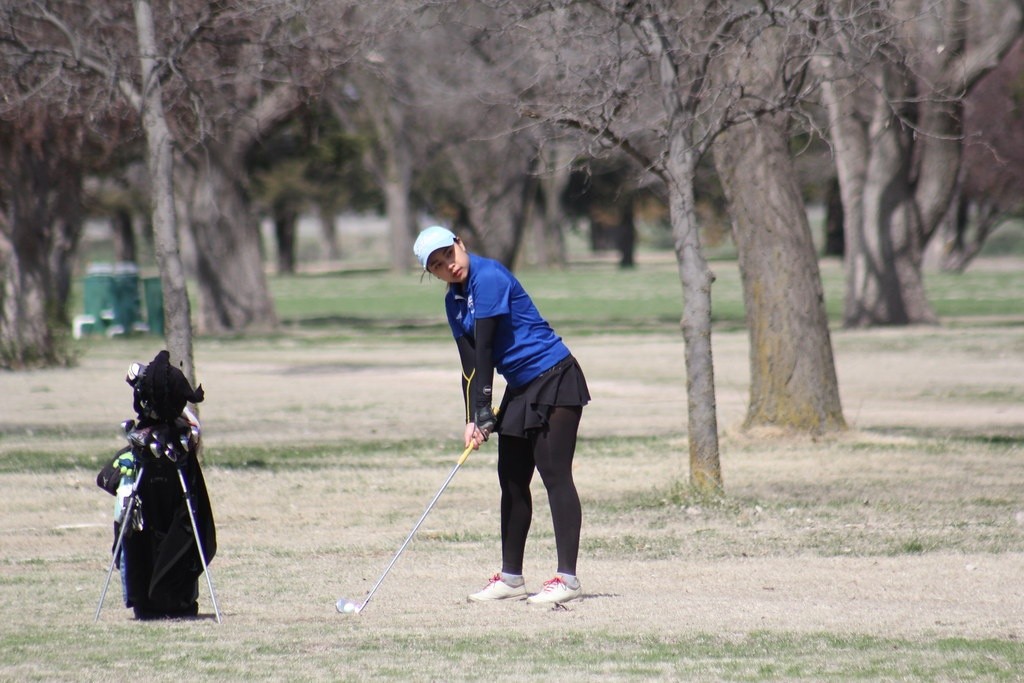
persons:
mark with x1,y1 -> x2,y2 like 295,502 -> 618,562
413,226 -> 593,607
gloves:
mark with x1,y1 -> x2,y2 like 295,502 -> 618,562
471,405 -> 496,442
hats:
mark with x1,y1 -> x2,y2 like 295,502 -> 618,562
413,226 -> 457,270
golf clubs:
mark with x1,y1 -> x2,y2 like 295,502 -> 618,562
114,419 -> 205,465
335,406 -> 500,616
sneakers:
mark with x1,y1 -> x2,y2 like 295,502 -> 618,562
468,574 -> 582,603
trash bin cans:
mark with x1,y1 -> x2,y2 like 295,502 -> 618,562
79,269 -> 165,341
